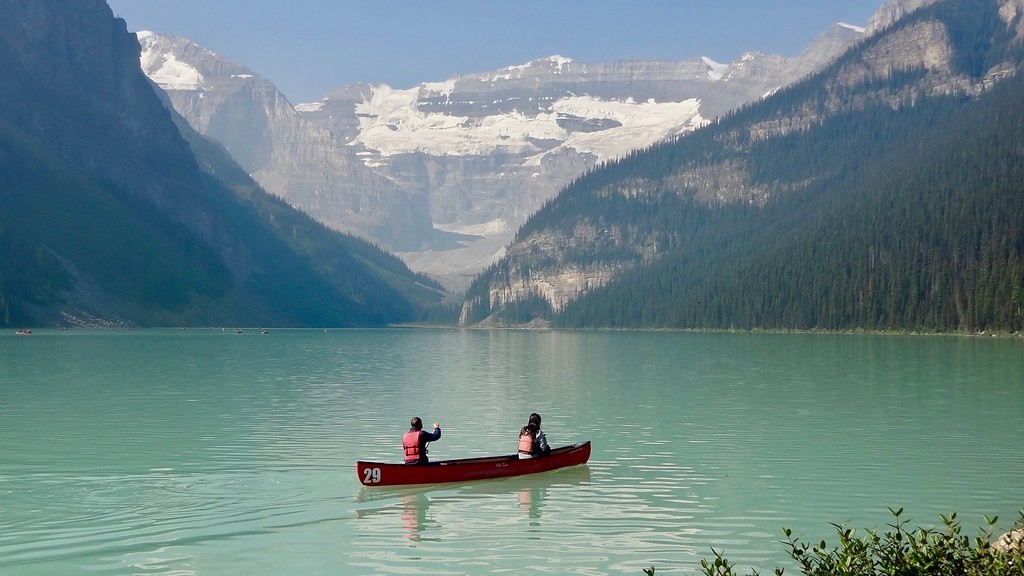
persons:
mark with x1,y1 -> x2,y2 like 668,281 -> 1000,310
402,417 -> 441,465
517,413 -> 550,459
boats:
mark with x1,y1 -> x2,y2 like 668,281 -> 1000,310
355,441 -> 592,487
